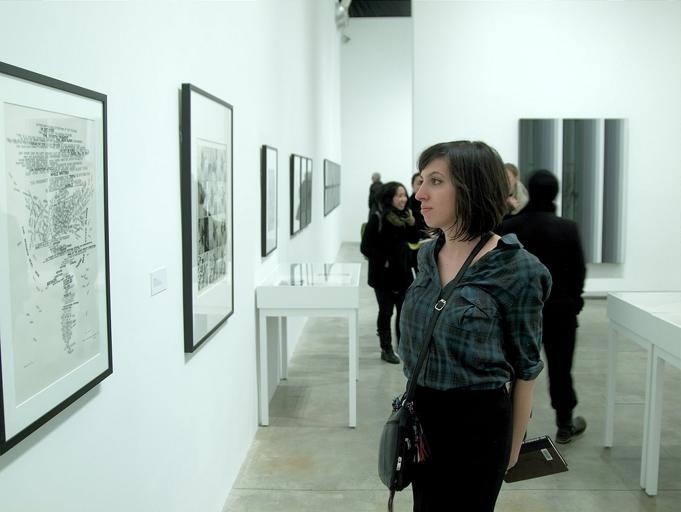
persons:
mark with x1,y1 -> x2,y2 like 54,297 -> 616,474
496,163 -> 586,444
360,173 -> 430,365
396,138 -> 553,511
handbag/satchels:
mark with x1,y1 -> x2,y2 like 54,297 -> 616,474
378,393 -> 423,491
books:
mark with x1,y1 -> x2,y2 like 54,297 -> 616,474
505,435 -> 569,483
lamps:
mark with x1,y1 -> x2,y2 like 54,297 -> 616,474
334,1 -> 354,47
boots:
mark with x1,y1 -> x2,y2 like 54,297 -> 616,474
556,411 -> 586,442
375,329 -> 400,364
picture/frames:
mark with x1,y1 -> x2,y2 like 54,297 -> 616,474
183,84 -> 236,353
261,144 -> 342,256
0,60 -> 113,455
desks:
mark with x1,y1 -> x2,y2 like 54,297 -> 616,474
645,313 -> 680,497
257,261 -> 363,426
604,288 -> 681,450
